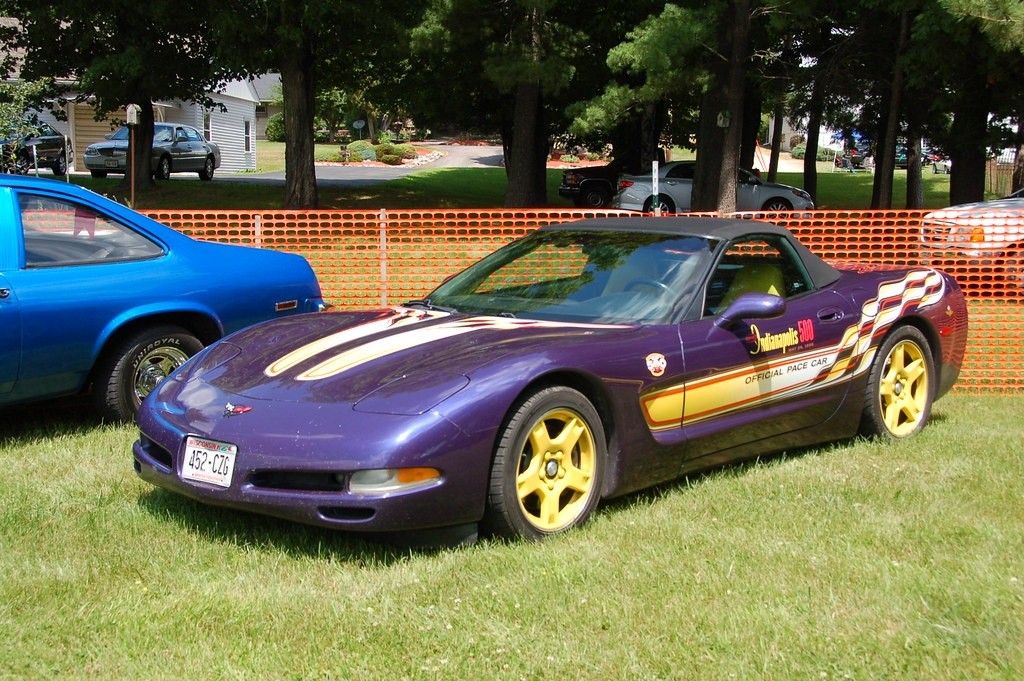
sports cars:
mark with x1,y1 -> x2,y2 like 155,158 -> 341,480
133,218 -> 968,548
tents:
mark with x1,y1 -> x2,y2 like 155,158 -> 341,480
831,129 -> 872,173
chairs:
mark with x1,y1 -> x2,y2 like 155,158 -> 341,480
714,262 -> 785,316
598,252 -> 659,299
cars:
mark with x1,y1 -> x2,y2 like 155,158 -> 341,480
612,159 -> 814,220
82,119 -> 222,184
914,188 -> 1024,301
0,172 -> 328,431
0,117 -> 74,178
931,156 -> 952,174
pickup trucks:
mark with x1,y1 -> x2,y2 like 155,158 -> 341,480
557,148 -> 761,208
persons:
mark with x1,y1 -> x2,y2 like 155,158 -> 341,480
339,145 -> 349,166
424,128 -> 431,140
842,154 -> 853,173
835,157 -> 839,166
